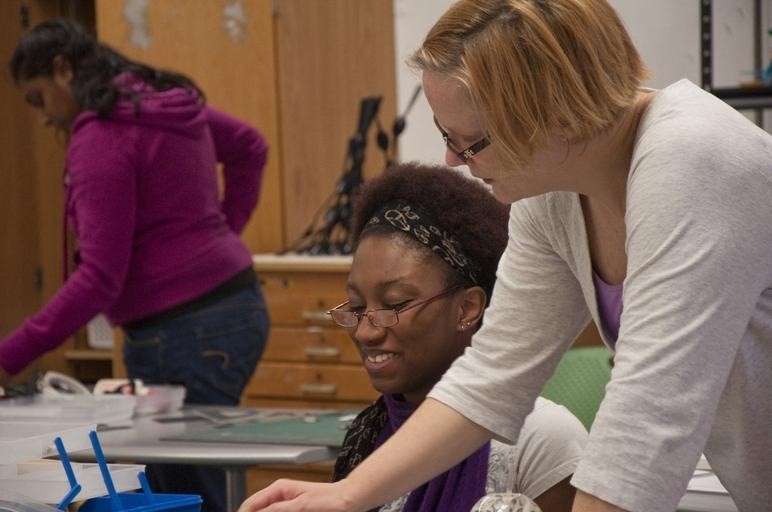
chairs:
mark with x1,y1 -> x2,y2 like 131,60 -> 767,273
114,257 -> 397,511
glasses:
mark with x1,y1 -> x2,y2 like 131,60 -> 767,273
326,282 -> 462,329
432,114 -> 495,164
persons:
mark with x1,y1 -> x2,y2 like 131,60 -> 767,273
0,13 -> 269,511
237,0 -> 772,512
328,159 -> 592,512
